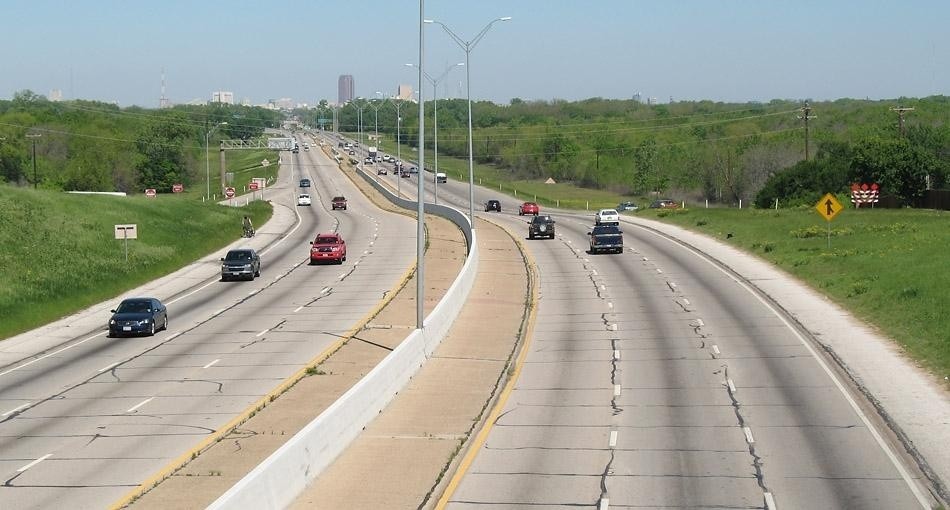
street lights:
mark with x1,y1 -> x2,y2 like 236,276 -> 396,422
25,133 -> 42,189
207,122 -> 227,200
344,97 -> 387,149
406,63 -> 465,205
376,91 -> 418,196
424,17 -> 511,228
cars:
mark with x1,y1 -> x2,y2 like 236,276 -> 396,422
339,137 -> 359,154
300,179 -> 310,187
588,225 -> 624,253
365,155 -> 418,177
519,202 -> 539,216
291,133 -> 317,153
595,209 -> 620,226
310,234 -> 346,264
617,201 -> 639,211
299,193 -> 311,206
221,249 -> 261,280
484,199 -> 501,212
109,298 -> 168,338
331,197 -> 347,209
648,200 -> 680,208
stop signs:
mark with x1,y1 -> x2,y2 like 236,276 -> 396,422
225,188 -> 235,198
146,189 -> 156,196
173,185 -> 182,193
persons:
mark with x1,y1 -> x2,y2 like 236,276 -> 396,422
241,216 -> 254,236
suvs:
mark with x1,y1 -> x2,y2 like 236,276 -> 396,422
527,216 -> 556,239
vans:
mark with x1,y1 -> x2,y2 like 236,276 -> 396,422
437,172 -> 446,183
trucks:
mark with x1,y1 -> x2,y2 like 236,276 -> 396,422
368,147 -> 377,158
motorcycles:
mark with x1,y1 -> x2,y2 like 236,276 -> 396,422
243,228 -> 255,237
